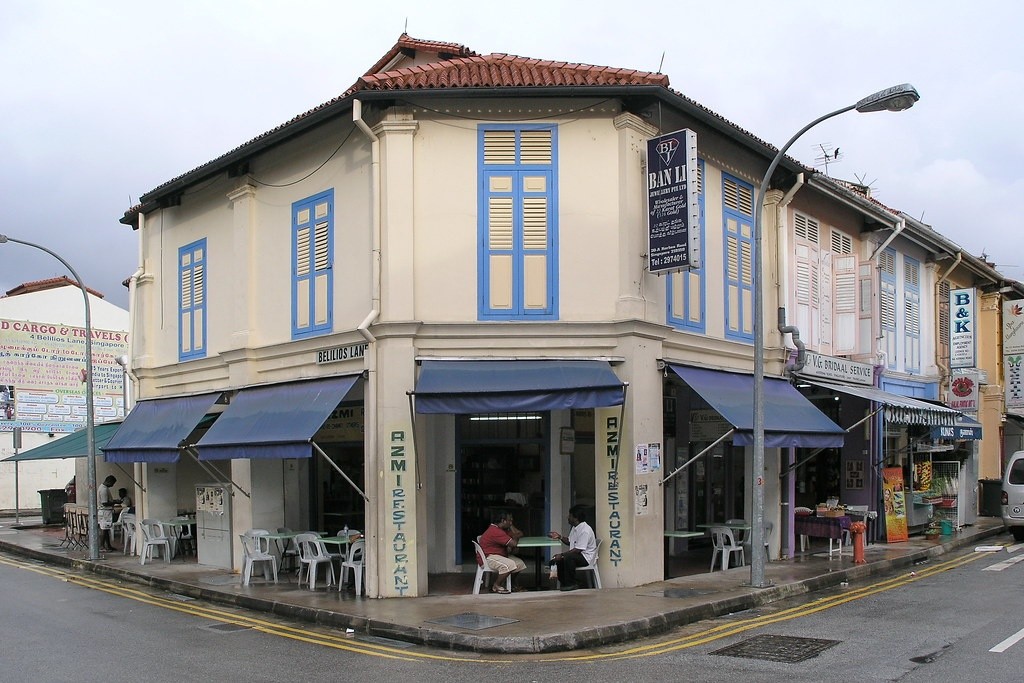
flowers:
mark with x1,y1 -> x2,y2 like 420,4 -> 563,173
924,522 -> 938,534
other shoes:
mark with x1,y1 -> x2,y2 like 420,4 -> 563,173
100,548 -> 112,552
560,586 -> 577,591
491,584 -> 510,594
107,546 -> 117,551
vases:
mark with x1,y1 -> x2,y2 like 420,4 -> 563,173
926,534 -> 939,540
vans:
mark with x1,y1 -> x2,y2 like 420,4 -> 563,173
998,450 -> 1024,543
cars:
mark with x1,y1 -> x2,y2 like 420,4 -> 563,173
63,477 -> 75,504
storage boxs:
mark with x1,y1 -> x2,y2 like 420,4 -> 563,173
505,492 -> 529,506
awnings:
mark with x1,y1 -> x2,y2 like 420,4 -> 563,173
191,369 -> 370,505
794,378 -> 963,467
405,359 -> 629,484
1,414 -> 216,461
660,365 -> 846,486
98,392 -> 234,496
910,398 -> 982,441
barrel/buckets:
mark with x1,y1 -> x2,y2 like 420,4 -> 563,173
939,520 -> 952,535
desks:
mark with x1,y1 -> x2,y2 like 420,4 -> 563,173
317,537 -> 365,583
696,524 -> 751,533
252,532 -> 328,580
664,530 -> 705,537
161,520 -> 197,558
516,535 -> 562,592
796,516 -> 851,556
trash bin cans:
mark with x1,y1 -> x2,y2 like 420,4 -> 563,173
978,479 -> 1002,517
37,489 -> 66,524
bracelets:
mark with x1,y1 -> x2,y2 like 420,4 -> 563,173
561,535 -> 563,539
561,554 -> 564,559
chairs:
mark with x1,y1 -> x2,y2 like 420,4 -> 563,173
139,519 -> 171,566
476,536 -> 490,587
168,524 -> 196,558
471,541 -> 511,594
294,534 -> 336,589
337,531 -> 364,564
120,513 -> 136,556
842,505 -> 868,547
707,519 -> 773,572
339,542 -> 365,596
238,528 -> 279,587
557,538 -> 604,591
273,528 -> 307,579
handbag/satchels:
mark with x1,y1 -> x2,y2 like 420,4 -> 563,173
549,562 -> 558,580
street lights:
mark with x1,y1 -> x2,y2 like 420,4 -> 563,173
744,81 -> 919,589
0,233 -> 105,561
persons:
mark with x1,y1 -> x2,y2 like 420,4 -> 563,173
113,488 -> 130,507
97,476 -> 117,551
476,510 -> 526,594
546,504 -> 595,591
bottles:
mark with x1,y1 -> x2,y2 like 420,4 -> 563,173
344,524 -> 349,540
